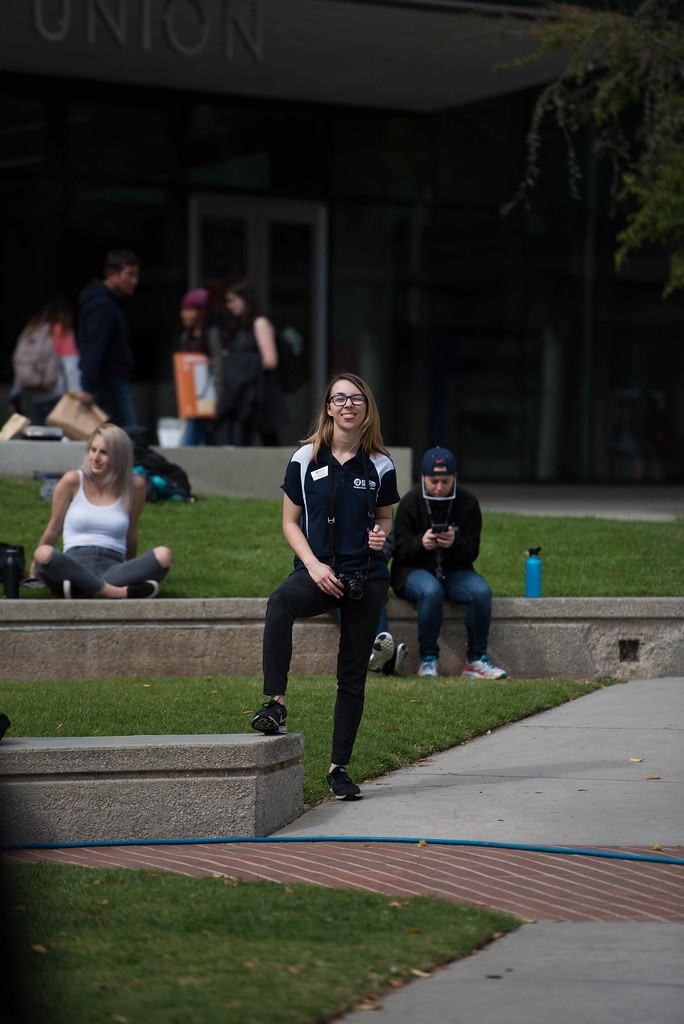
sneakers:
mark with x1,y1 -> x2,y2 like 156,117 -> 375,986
251,697 -> 288,734
417,656 -> 438,677
463,655 -> 507,680
327,766 -> 363,801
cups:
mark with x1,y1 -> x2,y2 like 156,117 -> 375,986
157,418 -> 182,448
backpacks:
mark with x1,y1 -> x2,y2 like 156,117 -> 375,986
13,322 -> 60,392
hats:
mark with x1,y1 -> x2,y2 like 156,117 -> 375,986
422,446 -> 457,476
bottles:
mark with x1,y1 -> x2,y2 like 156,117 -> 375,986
525,545 -> 542,598
3,550 -> 20,598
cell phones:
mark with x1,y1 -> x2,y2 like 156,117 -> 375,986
431,524 -> 449,535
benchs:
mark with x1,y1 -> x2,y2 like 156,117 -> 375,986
0,715 -> 316,853
0,590 -> 684,686
0,440 -> 416,508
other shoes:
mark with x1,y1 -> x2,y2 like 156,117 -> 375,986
62,579 -> 80,599
367,632 -> 394,671
390,643 -> 408,675
127,580 -> 159,598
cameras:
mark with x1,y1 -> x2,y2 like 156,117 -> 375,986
335,571 -> 367,600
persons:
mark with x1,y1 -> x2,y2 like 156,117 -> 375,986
157,282 -> 288,446
9,254 -> 138,425
24,425 -> 173,598
390,446 -> 506,679
335,513 -> 408,675
251,374 -> 401,801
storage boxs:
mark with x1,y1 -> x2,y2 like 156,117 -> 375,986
22,424 -> 69,441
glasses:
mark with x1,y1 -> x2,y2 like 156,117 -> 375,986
327,394 -> 366,406
